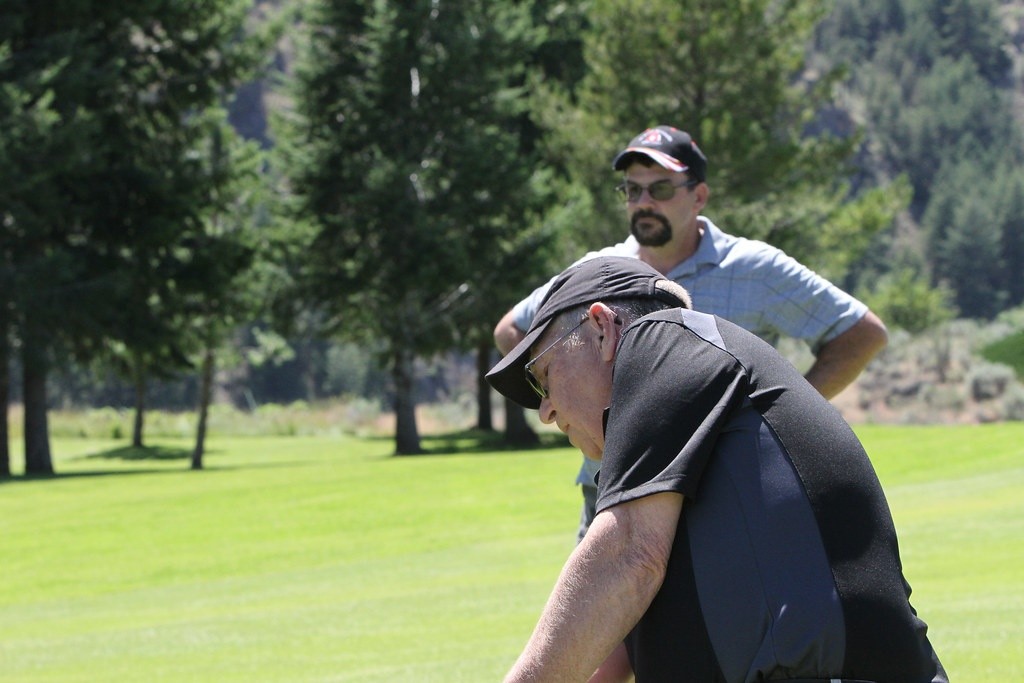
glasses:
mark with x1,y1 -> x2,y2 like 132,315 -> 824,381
615,178 -> 698,201
525,314 -> 623,398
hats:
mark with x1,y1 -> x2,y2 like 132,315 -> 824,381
485,256 -> 688,410
610,126 -> 709,182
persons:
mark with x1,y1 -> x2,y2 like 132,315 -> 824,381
485,258 -> 952,682
493,125 -> 888,542
